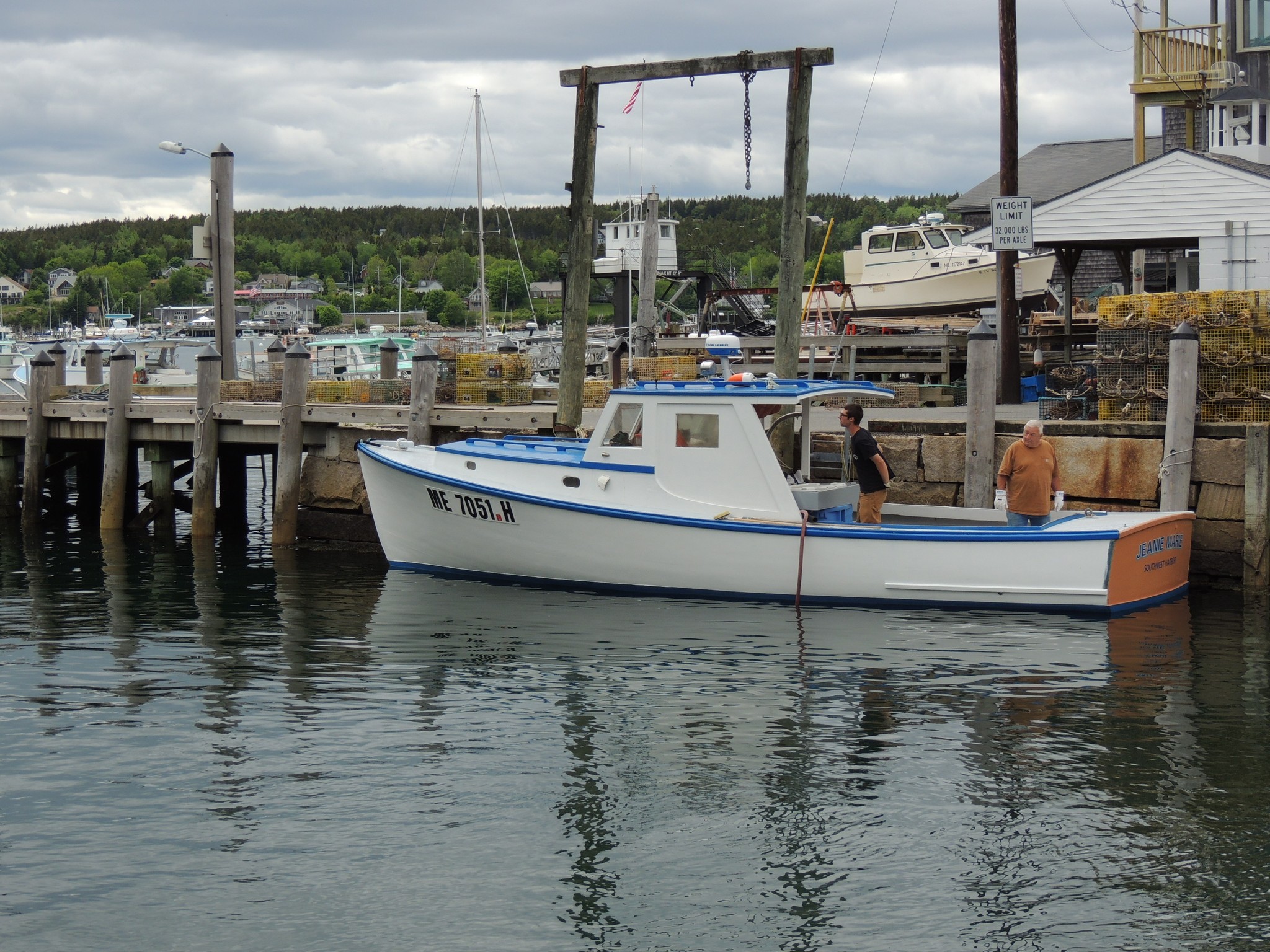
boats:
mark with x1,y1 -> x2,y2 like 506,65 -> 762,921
237,255 -> 417,380
237,327 -> 260,339
13,337 -> 215,397
403,330 -> 429,340
801,211 -> 1056,320
352,329 -> 1201,611
710,252 -> 770,319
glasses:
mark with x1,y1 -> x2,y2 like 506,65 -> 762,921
840,412 -> 851,418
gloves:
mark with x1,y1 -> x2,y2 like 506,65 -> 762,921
994,489 -> 1009,511
1054,491 -> 1063,512
883,477 -> 904,492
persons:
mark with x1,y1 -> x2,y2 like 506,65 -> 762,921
838,404 -> 904,524
994,419 -> 1064,527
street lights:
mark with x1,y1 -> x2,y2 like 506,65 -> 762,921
160,142 -> 238,380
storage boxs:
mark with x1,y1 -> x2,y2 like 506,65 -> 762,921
789,482 -> 860,511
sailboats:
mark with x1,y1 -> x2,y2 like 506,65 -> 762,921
411,89 -> 613,375
0,278 -> 154,379
257,301 -> 281,339
